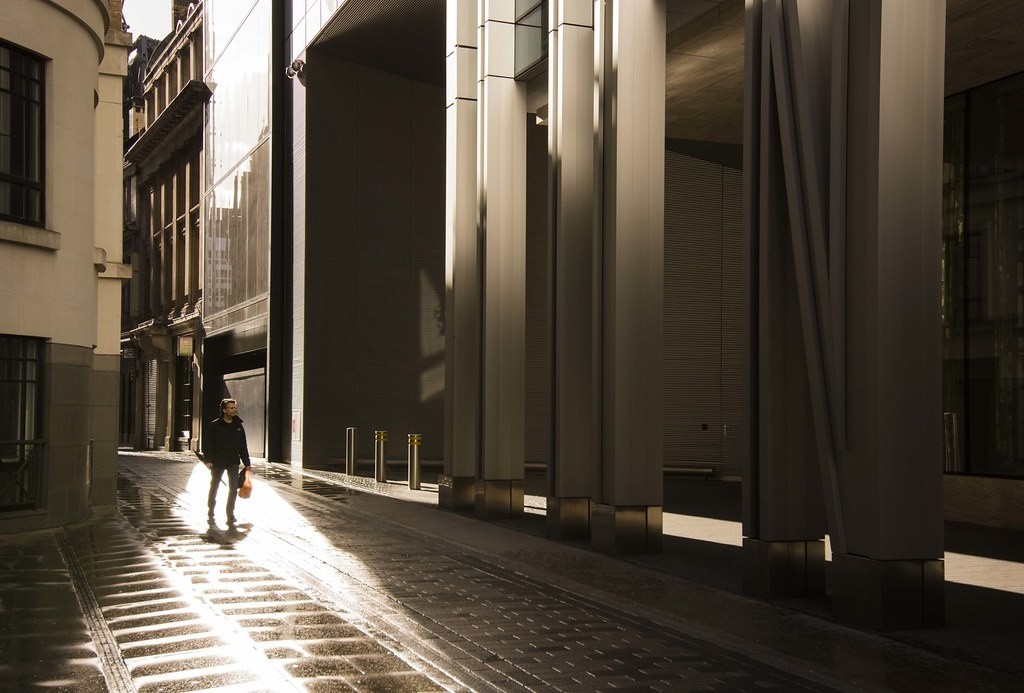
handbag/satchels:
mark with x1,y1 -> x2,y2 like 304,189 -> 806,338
239,468 -> 252,498
237,467 -> 247,488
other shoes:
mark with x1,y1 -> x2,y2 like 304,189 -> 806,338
228,516 -> 237,522
208,518 -> 215,525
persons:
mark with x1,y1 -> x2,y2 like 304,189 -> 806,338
201,397 -> 251,523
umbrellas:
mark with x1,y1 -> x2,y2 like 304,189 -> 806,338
192,449 -> 227,486
